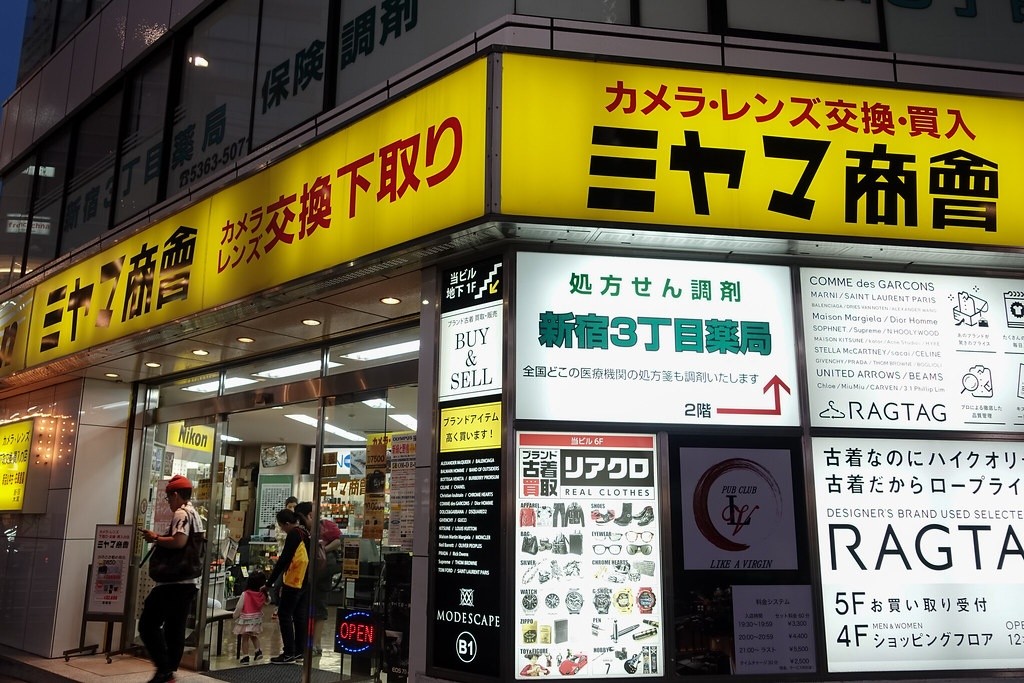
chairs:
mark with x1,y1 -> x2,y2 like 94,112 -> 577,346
344,561 -> 384,608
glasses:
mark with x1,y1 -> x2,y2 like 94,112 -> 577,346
592,544 -> 622,555
626,544 -> 653,555
592,531 -> 623,542
165,491 -> 179,501
625,531 -> 654,543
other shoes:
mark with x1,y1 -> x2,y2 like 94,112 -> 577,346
254,649 -> 263,661
296,654 -> 304,661
147,669 -> 171,683
591,510 -> 604,522
632,506 -> 651,520
638,508 -> 654,526
272,609 -> 278,619
240,656 -> 250,664
312,649 -> 322,657
271,654 -> 296,664
596,510 -> 615,526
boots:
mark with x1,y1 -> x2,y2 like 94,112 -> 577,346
618,503 -> 633,526
614,514 -> 622,523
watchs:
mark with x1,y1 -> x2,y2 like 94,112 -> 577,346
153,536 -> 159,545
520,584 -> 658,617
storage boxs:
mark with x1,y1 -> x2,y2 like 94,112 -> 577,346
221,465 -> 255,541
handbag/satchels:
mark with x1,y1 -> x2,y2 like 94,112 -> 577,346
522,534 -> 539,555
149,508 -> 208,583
283,528 -> 309,588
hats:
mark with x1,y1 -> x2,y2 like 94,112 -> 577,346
165,475 -> 193,492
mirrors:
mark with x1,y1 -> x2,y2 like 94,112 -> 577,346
259,446 -> 288,468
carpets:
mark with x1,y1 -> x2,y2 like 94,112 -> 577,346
198,663 -> 359,683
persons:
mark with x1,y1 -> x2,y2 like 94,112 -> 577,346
294,501 -> 342,657
286,496 -> 298,512
260,510 -> 311,665
139,475 -> 205,683
231,571 -> 271,665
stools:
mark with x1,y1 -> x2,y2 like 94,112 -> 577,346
184,606 -> 241,660
